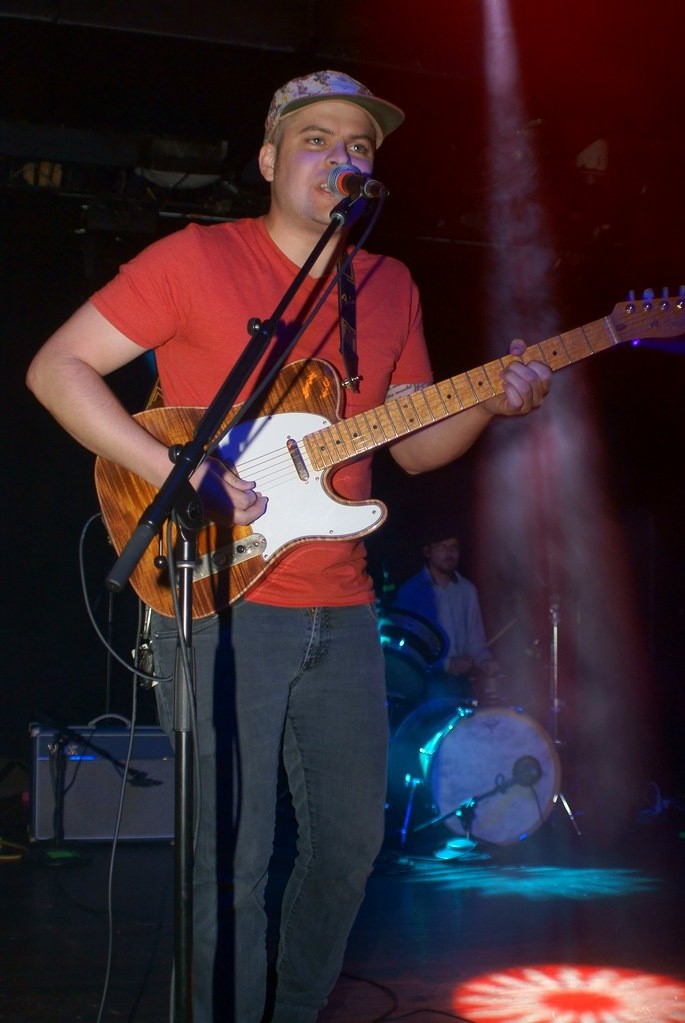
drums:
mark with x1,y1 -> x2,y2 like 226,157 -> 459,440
388,698 -> 563,847
381,607 -> 452,714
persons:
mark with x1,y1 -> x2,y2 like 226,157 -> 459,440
394,526 -> 504,698
27,70 -> 553,1023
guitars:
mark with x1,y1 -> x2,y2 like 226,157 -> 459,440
95,289 -> 684,618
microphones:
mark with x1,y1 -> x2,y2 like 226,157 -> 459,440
129,773 -> 146,786
324,165 -> 389,200
517,764 -> 539,785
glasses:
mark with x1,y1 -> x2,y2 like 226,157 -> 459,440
428,545 -> 460,552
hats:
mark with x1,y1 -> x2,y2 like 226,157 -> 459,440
422,521 -> 464,545
262,68 -> 407,151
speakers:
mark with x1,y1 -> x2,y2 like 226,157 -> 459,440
30,724 -> 178,847
